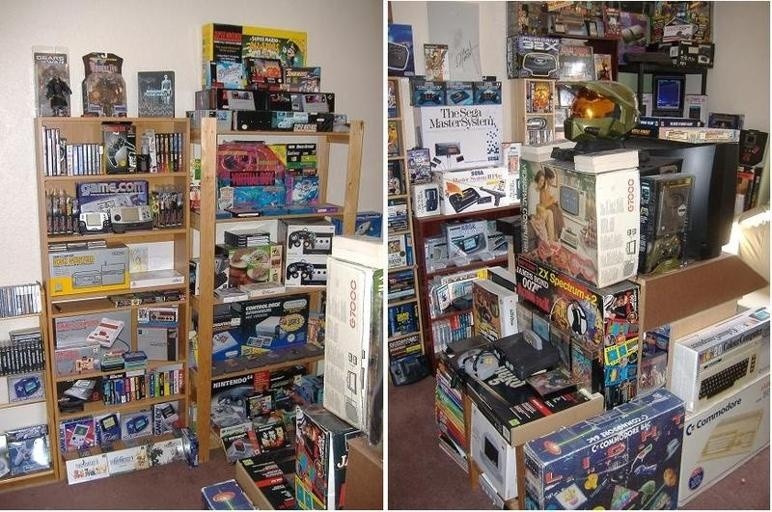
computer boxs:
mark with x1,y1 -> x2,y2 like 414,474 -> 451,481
641,172 -> 694,274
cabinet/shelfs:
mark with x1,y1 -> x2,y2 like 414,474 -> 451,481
509,38 -> 708,146
414,204 -> 522,378
191,116 -> 364,465
34,116 -> 191,483
0,286 -> 60,494
387,77 -> 426,375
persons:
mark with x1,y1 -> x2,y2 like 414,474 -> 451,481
526,166 -> 565,257
160,74 -> 173,104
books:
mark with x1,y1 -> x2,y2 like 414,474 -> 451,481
435,362 -> 469,475
101,368 -> 184,405
388,81 -> 423,365
430,312 -> 475,355
45,189 -> 78,235
156,133 -> 184,172
41,125 -> 104,176
0,284 -> 40,317
0,328 -> 46,376
152,191 -> 183,229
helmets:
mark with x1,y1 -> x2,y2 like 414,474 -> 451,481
555,78 -> 641,148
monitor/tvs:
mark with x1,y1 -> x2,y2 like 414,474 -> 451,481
625,138 -> 740,261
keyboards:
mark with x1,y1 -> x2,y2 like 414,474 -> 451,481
699,357 -> 749,399
387,42 -> 408,70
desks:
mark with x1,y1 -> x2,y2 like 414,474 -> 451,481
637,252 -> 738,344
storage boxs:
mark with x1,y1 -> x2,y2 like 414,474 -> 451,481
641,286 -> 770,509
49,243 -> 130,297
186,21 -> 349,132
409,105 -> 518,218
507,1 -> 713,83
